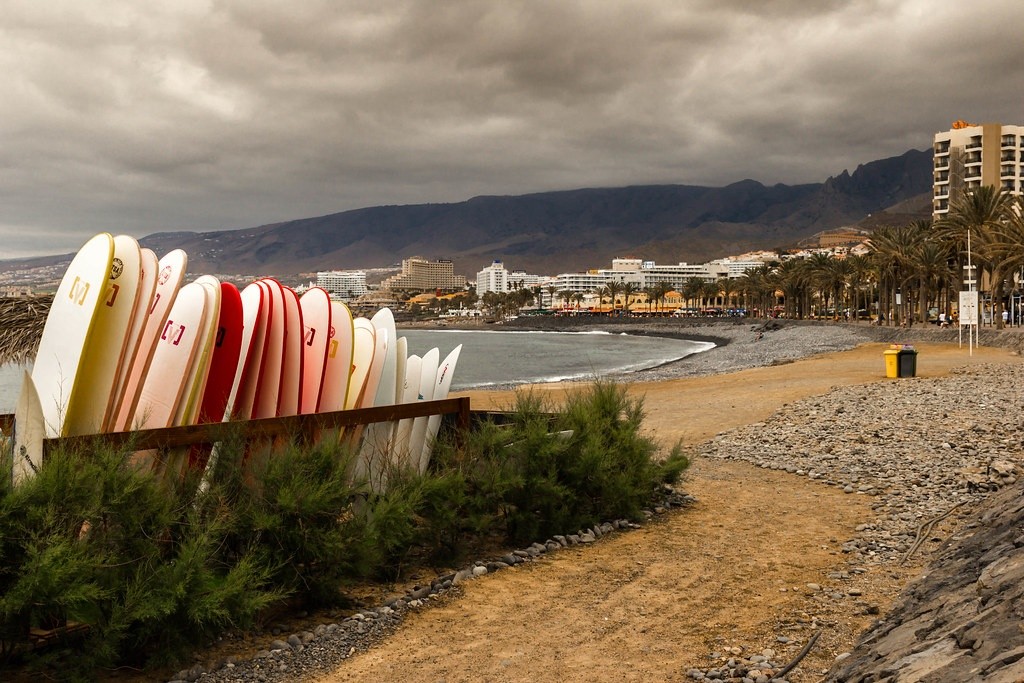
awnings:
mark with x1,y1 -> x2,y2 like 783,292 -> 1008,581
557,308 -> 718,313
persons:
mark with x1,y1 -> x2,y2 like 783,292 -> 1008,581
870,309 -> 959,328
844,308 -> 857,323
1002,309 -> 1008,327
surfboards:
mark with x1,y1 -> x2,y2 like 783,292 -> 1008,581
28,230 -> 464,500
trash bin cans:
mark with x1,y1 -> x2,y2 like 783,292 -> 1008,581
898,350 -> 919,377
890,344 -> 907,351
884,349 -> 900,378
